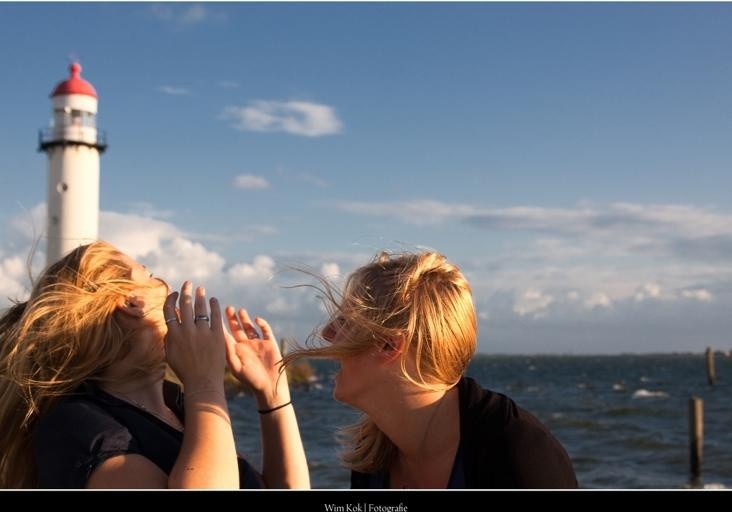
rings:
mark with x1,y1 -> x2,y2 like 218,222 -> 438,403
196,315 -> 209,320
249,333 -> 259,338
166,317 -> 177,324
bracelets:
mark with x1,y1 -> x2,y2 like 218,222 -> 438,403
258,400 -> 294,414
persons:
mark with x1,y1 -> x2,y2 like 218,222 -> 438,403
321,252 -> 578,489
0,240 -> 310,489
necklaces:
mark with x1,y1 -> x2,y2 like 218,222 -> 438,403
105,386 -> 185,431
